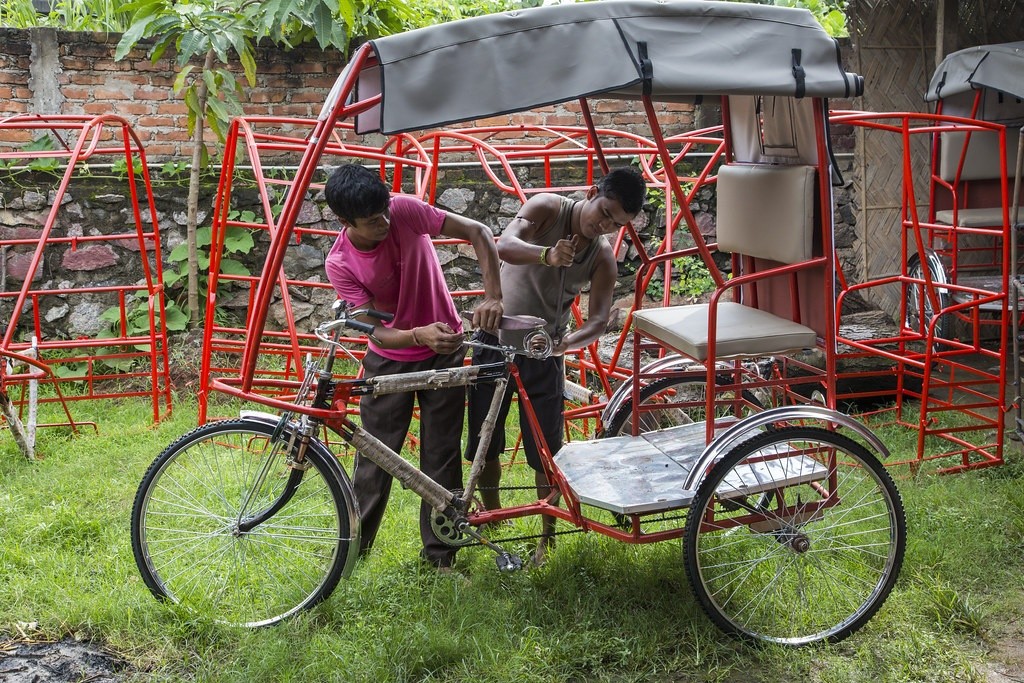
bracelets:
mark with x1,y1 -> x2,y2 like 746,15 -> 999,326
411,326 -> 422,346
539,245 -> 553,267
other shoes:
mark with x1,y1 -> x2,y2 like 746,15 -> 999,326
436,567 -> 465,581
527,546 -> 550,568
476,520 -> 513,537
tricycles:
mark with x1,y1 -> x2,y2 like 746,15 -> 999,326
128,0 -> 909,652
905,40 -> 1024,374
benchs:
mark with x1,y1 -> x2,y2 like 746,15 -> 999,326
629,163 -> 820,477
933,126 -> 1024,297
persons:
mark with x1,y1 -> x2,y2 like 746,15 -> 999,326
324,163 -> 504,590
464,167 -> 646,570
